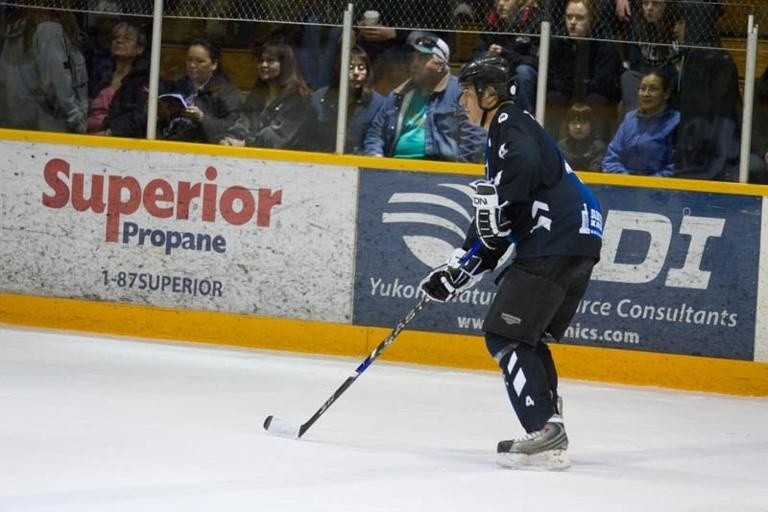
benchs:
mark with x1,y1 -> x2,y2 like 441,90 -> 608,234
714,28 -> 767,99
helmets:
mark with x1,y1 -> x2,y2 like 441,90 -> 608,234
458,53 -> 518,112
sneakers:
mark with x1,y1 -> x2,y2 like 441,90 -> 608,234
499,417 -> 568,453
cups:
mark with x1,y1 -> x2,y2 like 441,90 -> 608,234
363,10 -> 381,29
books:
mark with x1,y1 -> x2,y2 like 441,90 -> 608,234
142,87 -> 186,113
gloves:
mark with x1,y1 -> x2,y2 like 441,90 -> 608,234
420,248 -> 484,304
470,179 -> 512,252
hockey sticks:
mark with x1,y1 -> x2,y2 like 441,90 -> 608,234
264,242 -> 481,441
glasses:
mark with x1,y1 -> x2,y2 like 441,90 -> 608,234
416,37 -> 448,58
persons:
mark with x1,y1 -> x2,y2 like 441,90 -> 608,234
300,0 -> 485,162
219,31 -> 321,153
418,51 -> 605,454
5,2 -> 149,137
143,38 -> 242,142
477,0 -> 768,183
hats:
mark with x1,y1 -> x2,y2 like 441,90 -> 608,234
400,36 -> 450,63
